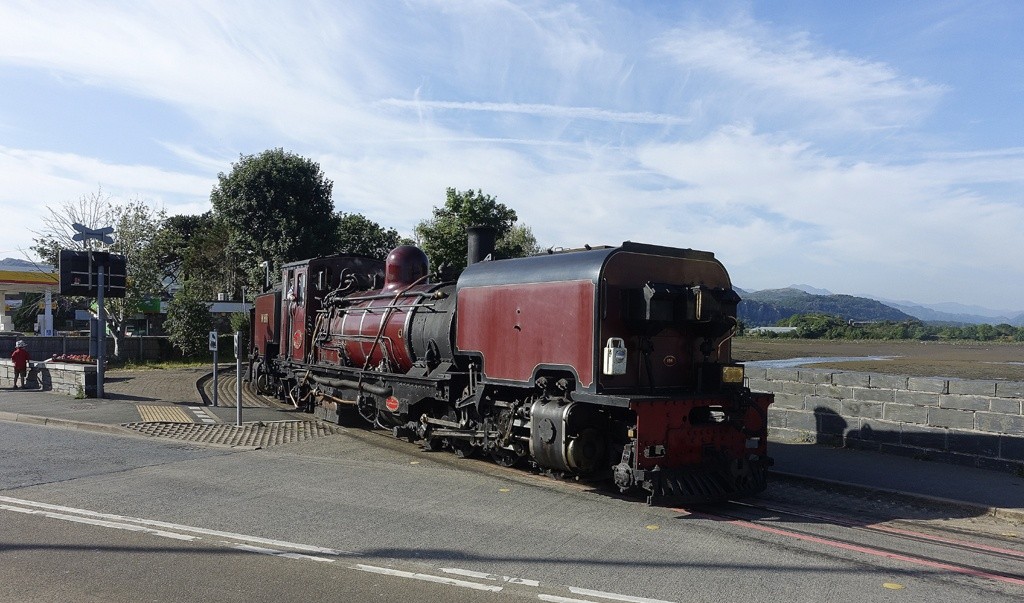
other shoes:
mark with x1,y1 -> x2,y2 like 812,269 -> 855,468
13,385 -> 19,389
21,386 -> 28,389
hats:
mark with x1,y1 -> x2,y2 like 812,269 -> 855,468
15,340 -> 28,348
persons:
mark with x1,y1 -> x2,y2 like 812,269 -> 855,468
287,275 -> 301,300
11,340 -> 30,389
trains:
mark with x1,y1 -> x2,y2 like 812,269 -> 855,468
248,224 -> 775,506
